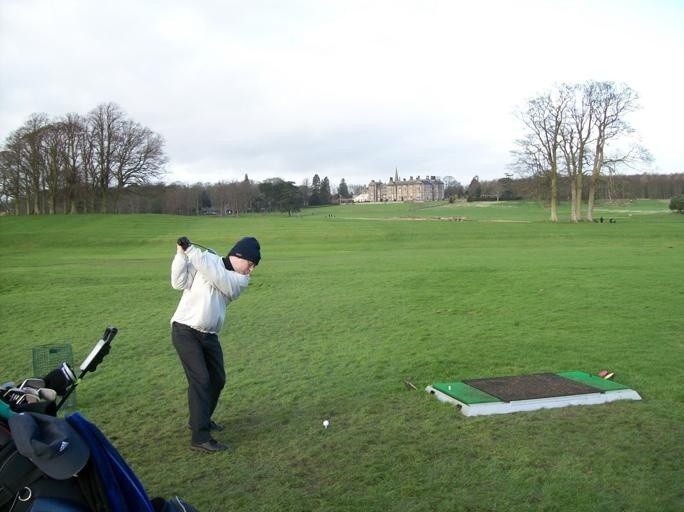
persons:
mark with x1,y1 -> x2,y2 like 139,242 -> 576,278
169,234 -> 260,452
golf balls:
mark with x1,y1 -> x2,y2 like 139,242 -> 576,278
323,420 -> 329,425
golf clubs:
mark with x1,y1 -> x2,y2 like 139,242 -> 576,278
189,239 -> 218,254
2,377 -> 58,407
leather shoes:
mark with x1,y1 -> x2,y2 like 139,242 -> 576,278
187,421 -> 224,432
190,438 -> 231,454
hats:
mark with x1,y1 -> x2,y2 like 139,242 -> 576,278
228,237 -> 261,265
8,411 -> 90,480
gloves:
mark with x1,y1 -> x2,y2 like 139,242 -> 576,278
177,237 -> 191,251
